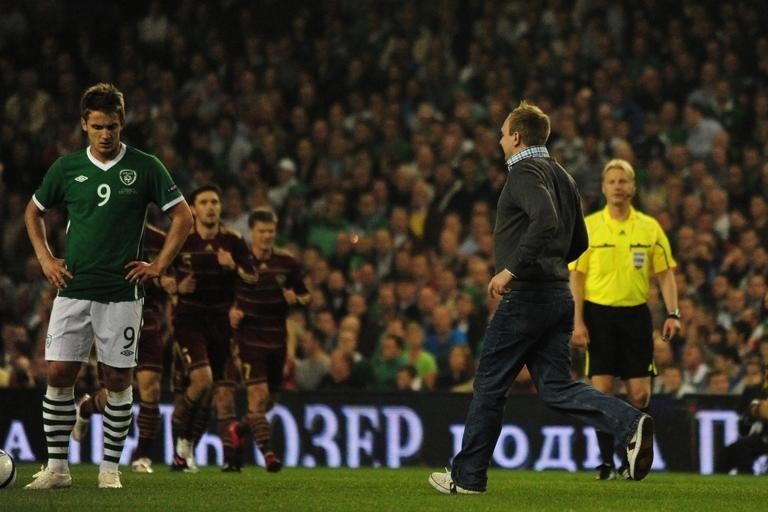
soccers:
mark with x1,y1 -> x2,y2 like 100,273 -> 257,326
0,449 -> 16,486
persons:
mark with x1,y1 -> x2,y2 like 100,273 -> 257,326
0,0 -> 767,474
429,97 -> 655,493
24,85 -> 194,489
568,161 -> 681,482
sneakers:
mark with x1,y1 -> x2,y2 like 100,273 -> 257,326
97,468 -> 123,488
229,421 -> 244,449
172,437 -> 199,473
429,472 -> 486,494
131,457 -> 153,473
25,465 -> 72,489
72,393 -> 91,440
596,414 -> 654,481
265,455 -> 282,472
